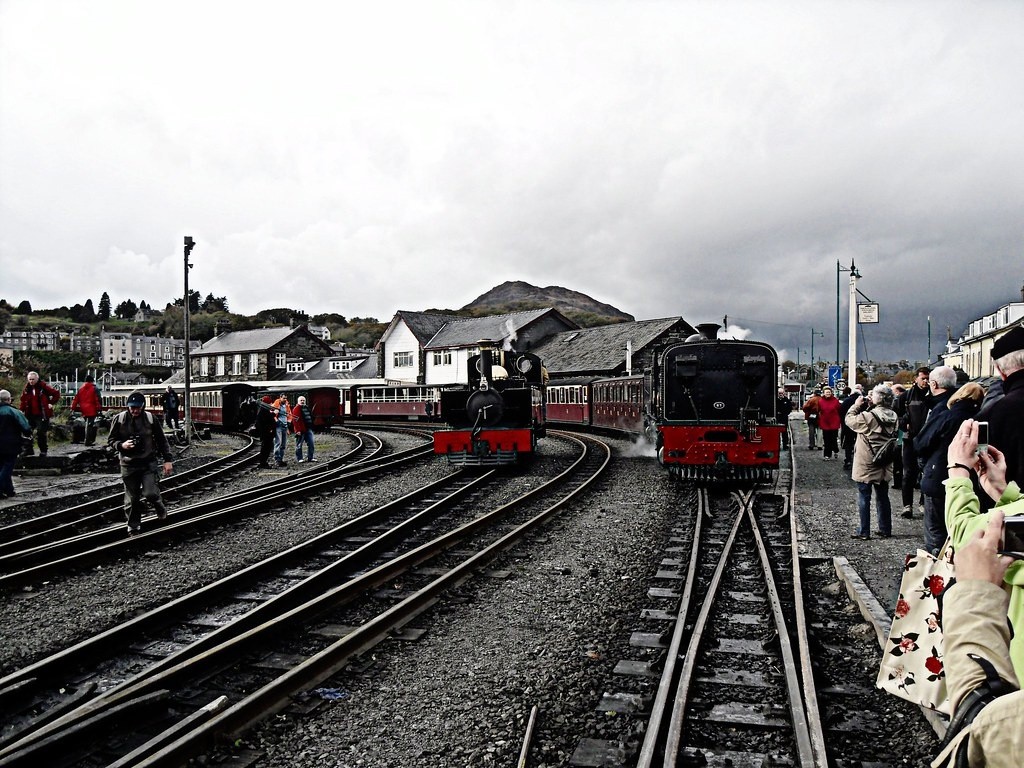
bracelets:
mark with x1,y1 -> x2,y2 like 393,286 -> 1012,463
946,463 -> 971,474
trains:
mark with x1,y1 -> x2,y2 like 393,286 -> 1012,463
60,383 -> 256,429
338,338 -> 544,468
542,323 -> 786,482
254,385 -> 341,430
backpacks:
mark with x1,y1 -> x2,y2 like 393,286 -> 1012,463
862,411 -> 900,468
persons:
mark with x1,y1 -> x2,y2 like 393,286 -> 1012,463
108,391 -> 173,536
845,384 -> 899,540
19,371 -> 60,456
256,396 -> 278,469
802,326 -> 1024,768
70,375 -> 102,446
0,389 -> 31,500
160,386 -> 180,429
292,396 -> 317,463
270,393 -> 293,467
776,388 -> 792,450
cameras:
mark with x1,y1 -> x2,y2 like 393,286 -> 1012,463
863,397 -> 867,402
976,421 -> 989,456
1001,516 -> 1024,558
130,435 -> 140,444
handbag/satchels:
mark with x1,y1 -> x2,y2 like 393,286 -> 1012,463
875,535 -> 958,716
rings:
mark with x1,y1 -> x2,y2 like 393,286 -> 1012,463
961,434 -> 970,437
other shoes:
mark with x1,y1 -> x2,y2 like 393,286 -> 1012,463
261,464 -> 272,469
311,459 -> 318,462
875,531 -> 892,537
127,526 -> 141,537
834,453 -> 839,459
158,510 -> 167,520
299,460 -> 304,463
20,450 -> 34,456
275,461 -> 287,467
823,456 -> 831,461
901,505 -> 912,517
85,443 -> 95,446
0,491 -> 16,499
917,504 -> 925,516
850,533 -> 870,540
40,452 -> 46,456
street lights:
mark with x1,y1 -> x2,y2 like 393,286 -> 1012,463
184,236 -> 196,439
797,348 -> 806,379
811,327 -> 824,390
836,258 -> 862,365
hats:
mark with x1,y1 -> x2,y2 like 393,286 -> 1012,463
990,326 -> 1024,359
262,396 -> 272,404
127,393 -> 145,407
